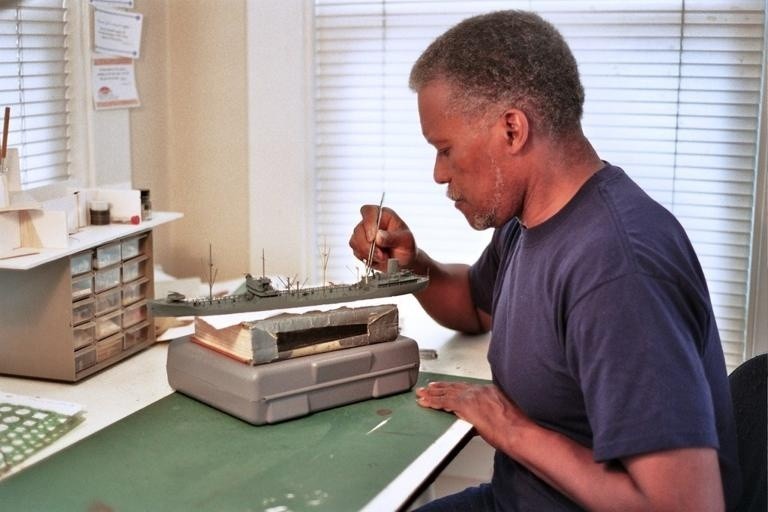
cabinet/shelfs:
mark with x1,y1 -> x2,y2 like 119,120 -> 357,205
1,186 -> 186,382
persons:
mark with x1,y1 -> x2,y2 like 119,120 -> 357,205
350,11 -> 742,512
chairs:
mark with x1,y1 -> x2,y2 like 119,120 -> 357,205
728,352 -> 767,511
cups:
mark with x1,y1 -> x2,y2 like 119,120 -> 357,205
89,201 -> 110,223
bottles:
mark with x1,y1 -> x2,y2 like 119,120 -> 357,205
139,186 -> 153,222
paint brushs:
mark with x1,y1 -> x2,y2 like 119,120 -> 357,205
1,107 -> 10,173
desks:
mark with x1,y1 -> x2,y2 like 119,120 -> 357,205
0,293 -> 497,512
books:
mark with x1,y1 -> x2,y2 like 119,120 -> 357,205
190,304 -> 399,367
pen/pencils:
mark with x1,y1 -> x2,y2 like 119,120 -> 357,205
418,349 -> 438,360
